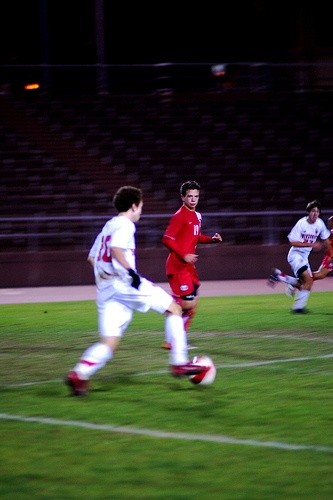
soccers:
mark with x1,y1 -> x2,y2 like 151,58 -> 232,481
187,355 -> 216,386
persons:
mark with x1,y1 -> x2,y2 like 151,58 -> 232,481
268,200 -> 333,315
162,180 -> 222,350
287,216 -> 333,300
65,187 -> 204,395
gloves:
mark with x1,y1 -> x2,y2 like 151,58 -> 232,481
129,269 -> 141,288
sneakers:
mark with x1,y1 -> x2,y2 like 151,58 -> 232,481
171,362 -> 206,377
66,371 -> 88,396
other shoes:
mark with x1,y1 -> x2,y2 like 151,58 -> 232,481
293,308 -> 311,315
268,267 -> 280,285
161,342 -> 172,349
